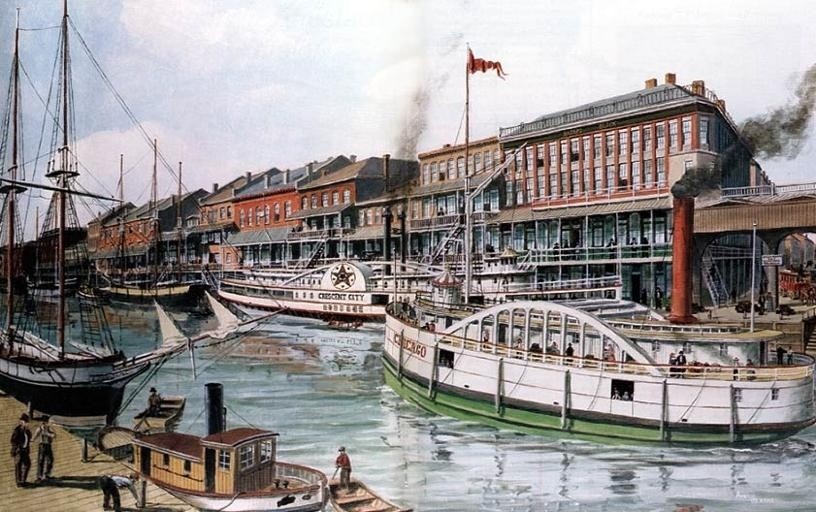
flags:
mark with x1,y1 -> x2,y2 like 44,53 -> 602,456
467,46 -> 509,81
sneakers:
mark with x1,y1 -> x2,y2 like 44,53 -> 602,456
103,506 -> 111,511
17,481 -> 33,488
45,475 -> 56,480
34,478 -> 41,484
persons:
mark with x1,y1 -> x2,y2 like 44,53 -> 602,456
605,237 -> 649,259
777,344 -> 794,365
10,413 -> 57,487
134,388 -> 162,419
335,446 -> 351,492
99,472 -> 142,512
669,351 -> 686,379
514,338 -> 574,366
552,240 -> 581,261
480,327 -> 489,349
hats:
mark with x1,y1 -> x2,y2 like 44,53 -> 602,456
338,447 -> 345,451
149,387 -> 157,393
40,415 -> 50,422
19,412 -> 29,423
